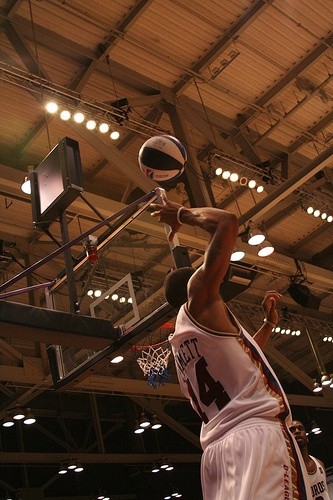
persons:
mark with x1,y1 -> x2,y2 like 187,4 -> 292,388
288,420 -> 330,500
149,195 -> 313,500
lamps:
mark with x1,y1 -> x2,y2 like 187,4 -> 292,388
2,97 -> 333,500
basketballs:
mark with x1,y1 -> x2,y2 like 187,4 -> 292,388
138,134 -> 187,183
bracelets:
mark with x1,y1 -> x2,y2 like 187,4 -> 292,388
264,319 -> 276,327
177,207 -> 184,225
